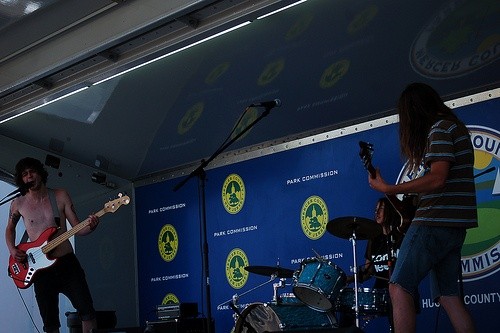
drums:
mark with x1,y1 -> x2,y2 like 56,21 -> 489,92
292,258 -> 348,313
232,303 -> 339,333
338,287 -> 392,315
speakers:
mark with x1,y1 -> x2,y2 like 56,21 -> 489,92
147,318 -> 215,333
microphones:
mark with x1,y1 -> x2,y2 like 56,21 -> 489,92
18,180 -> 37,192
250,99 -> 282,108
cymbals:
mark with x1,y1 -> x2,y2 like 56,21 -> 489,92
244,266 -> 295,279
325,216 -> 375,241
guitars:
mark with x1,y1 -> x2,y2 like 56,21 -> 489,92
8,192 -> 130,290
358,140 -> 420,236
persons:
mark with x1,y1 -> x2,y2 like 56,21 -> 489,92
347,196 -> 423,318
369,82 -> 479,333
5,157 -> 100,332
